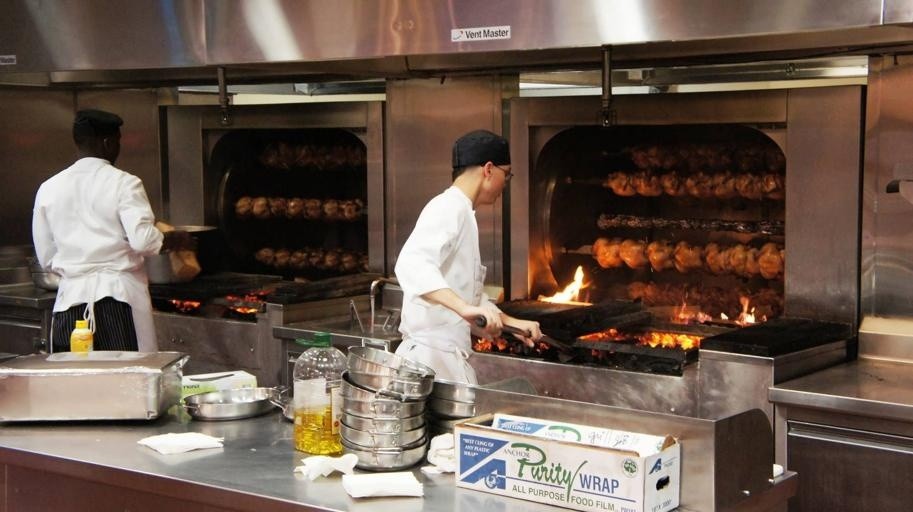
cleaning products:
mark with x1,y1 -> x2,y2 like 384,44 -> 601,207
70,319 -> 94,356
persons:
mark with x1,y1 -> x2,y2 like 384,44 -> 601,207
394,130 -> 543,385
32,110 -> 191,353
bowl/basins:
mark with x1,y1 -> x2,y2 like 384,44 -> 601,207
31,263 -> 63,292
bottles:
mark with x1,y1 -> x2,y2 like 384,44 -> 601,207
68,319 -> 95,358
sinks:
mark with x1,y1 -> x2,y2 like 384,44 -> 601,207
317,316 -> 402,332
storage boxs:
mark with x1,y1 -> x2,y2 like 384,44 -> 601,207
451,413 -> 680,511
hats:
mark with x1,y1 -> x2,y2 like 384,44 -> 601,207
452,129 -> 512,166
74,108 -> 124,128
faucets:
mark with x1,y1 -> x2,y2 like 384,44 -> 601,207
370,278 -> 399,332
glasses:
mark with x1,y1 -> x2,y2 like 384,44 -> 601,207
495,166 -> 514,181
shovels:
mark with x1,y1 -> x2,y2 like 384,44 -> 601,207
476,315 -> 588,363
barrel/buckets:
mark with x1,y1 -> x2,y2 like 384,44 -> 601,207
293,332 -> 348,453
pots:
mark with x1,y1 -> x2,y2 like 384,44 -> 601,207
159,225 -> 218,253
181,382 -> 289,422
339,343 -> 432,474
